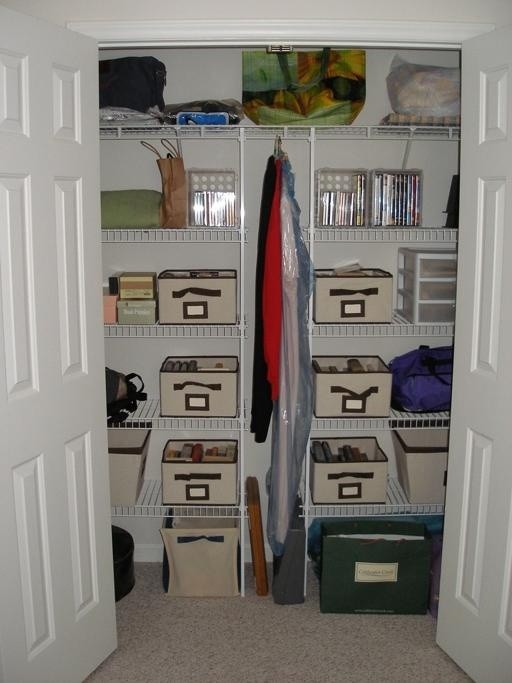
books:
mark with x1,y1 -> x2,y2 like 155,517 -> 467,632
165,360 -> 225,370
166,443 -> 234,463
310,440 -> 368,462
188,188 -> 237,228
311,358 -> 382,373
316,171 -> 419,226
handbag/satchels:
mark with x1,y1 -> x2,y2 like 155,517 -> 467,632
320,521 -> 431,615
99,56 -> 166,114
157,157 -> 187,230
242,49 -> 366,129
391,345 -> 453,415
164,99 -> 245,126
273,497 -> 304,605
